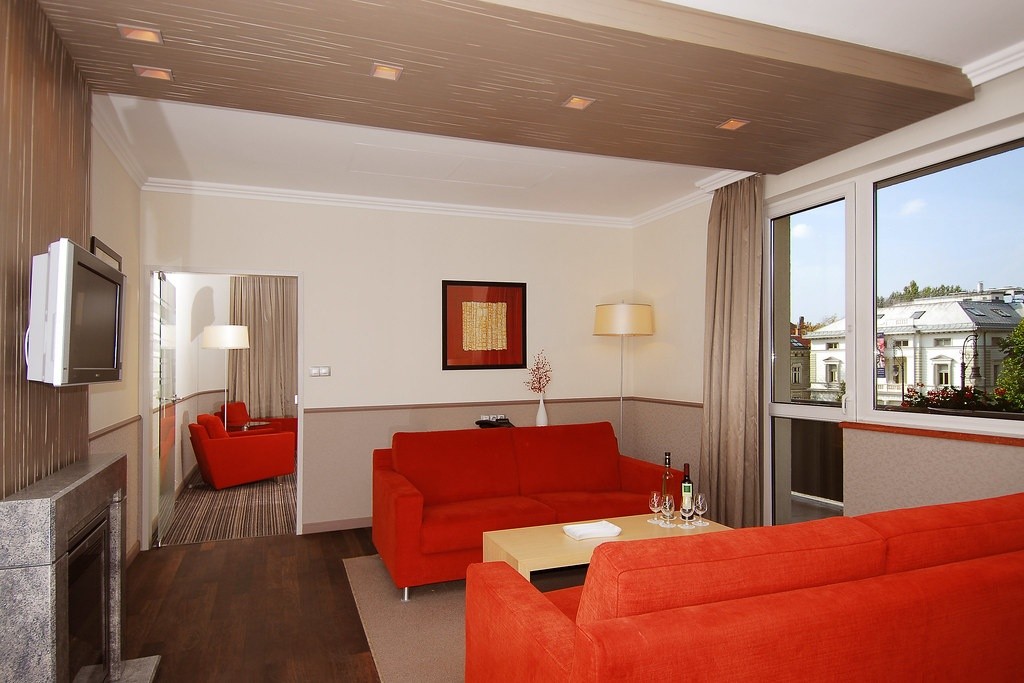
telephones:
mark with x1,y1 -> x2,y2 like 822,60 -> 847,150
475,419 -> 515,428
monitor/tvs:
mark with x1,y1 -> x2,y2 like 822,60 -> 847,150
24,238 -> 127,387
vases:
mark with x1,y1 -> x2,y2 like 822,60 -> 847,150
536,391 -> 550,426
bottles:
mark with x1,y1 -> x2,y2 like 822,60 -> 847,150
681,464 -> 695,520
661,451 -> 676,505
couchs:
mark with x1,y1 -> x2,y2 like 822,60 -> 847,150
465,493 -> 1024,683
214,402 -> 298,448
188,413 -> 296,491
371,421 -> 686,603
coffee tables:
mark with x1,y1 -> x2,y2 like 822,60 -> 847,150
482,512 -> 734,584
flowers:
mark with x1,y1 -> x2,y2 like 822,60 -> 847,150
901,381 -> 1007,411
522,348 -> 552,393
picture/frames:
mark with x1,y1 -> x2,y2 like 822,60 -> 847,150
440,280 -> 528,365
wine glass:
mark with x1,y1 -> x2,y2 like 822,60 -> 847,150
678,494 -> 696,529
659,494 -> 677,527
693,493 -> 710,526
648,491 -> 663,525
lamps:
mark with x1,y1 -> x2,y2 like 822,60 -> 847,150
202,325 -> 251,431
593,299 -> 654,451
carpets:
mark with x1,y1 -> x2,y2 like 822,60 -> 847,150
342,553 -> 591,683
150,470 -> 296,548
